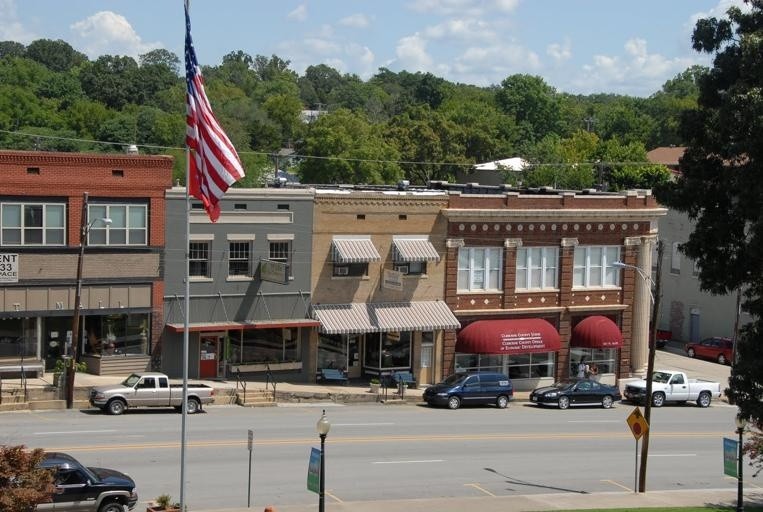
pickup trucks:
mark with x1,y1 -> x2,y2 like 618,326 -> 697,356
623,369 -> 723,408
88,371 -> 216,414
0,452 -> 138,512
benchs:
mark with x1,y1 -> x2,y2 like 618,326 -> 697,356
391,373 -> 417,389
319,367 -> 349,385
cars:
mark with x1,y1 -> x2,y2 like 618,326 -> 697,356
530,378 -> 622,409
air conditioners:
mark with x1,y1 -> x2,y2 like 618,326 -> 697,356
334,267 -> 349,275
394,265 -> 409,275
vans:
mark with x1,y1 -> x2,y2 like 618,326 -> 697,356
684,336 -> 736,366
423,372 -> 514,409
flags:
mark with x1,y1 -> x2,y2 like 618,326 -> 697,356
182,2 -> 245,223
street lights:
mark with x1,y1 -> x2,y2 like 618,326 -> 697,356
316,409 -> 330,512
734,412 -> 746,512
613,261 -> 657,493
61,192 -> 113,410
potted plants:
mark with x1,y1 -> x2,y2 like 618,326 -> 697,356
397,380 -> 409,394
52,359 -> 89,387
369,379 -> 382,393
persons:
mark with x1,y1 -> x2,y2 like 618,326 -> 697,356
590,363 -> 598,376
576,357 -> 585,377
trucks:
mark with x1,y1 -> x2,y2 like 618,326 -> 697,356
649,320 -> 673,349
